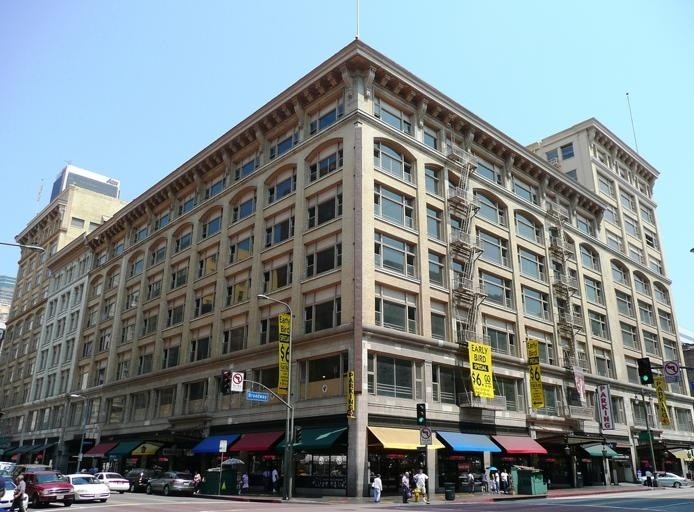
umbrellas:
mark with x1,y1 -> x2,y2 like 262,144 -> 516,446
221,458 -> 245,464
486,466 -> 498,470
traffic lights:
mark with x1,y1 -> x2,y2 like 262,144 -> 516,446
634,356 -> 655,386
221,367 -> 232,393
415,402 -> 426,426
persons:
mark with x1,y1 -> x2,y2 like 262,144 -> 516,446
272,466 -> 279,493
468,471 -> 475,493
645,469 -> 653,487
371,474 -> 383,502
10,475 -> 26,512
548,478 -> 552,489
81,465 -> 114,475
402,468 -> 429,503
237,472 -> 249,495
481,469 -> 509,494
193,471 -> 201,494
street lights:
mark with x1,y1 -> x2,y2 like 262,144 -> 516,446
67,389 -> 89,475
255,291 -> 294,499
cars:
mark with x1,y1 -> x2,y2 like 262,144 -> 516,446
145,470 -> 196,495
123,467 -> 160,492
638,469 -> 690,489
14,470 -> 74,508
57,473 -> 110,504
9,463 -> 53,480
93,471 -> 130,494
0,461 -> 30,512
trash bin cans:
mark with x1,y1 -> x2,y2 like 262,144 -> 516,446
444,482 -> 455,500
368,483 -> 374,496
